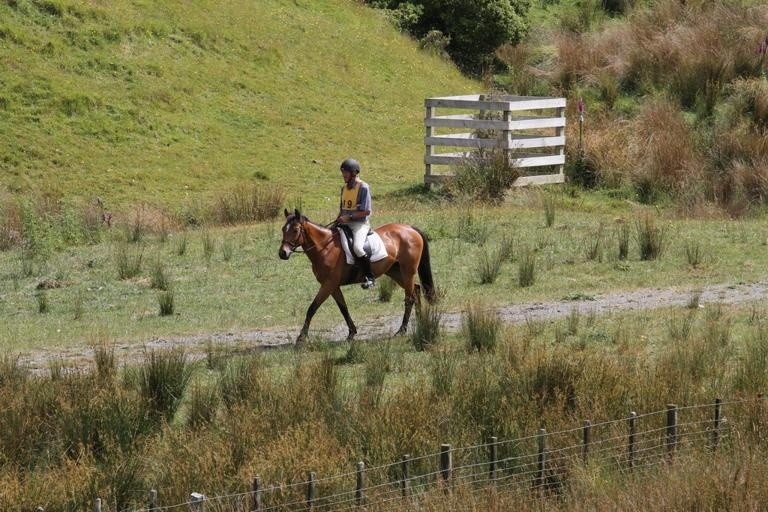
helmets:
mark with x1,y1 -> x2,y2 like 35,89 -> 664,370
341,159 -> 359,174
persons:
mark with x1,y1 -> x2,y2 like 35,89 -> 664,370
336,158 -> 377,290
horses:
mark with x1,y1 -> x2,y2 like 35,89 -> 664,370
279,209 -> 440,350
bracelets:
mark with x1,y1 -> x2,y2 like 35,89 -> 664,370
349,212 -> 353,221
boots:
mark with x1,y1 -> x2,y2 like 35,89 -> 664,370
357,252 -> 377,288
